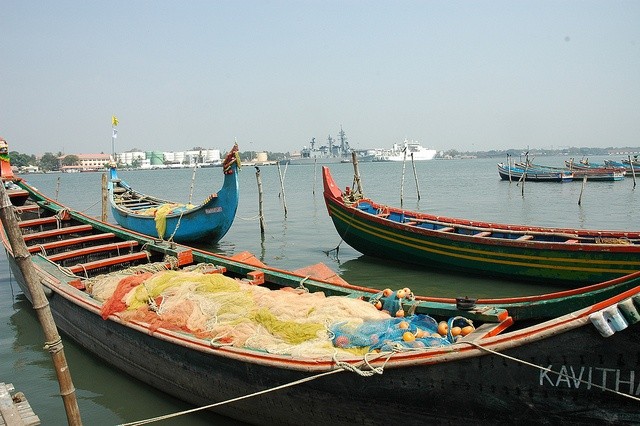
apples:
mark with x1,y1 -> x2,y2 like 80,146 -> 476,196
370,334 -> 379,345
383,288 -> 393,297
404,287 -> 410,294
395,310 -> 405,317
335,337 -> 348,347
396,290 -> 405,299
403,331 -> 415,341
423,331 -> 430,338
399,321 -> 410,328
462,325 -> 474,336
438,324 -> 449,335
451,326 -> 461,336
432,333 -> 442,338
374,300 -> 382,310
414,328 -> 423,338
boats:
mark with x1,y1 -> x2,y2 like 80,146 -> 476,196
565,156 -> 626,176
621,155 -> 640,167
515,153 -> 625,181
0,139 -> 640,426
106,139 -> 240,245
321,167 -> 639,285
604,155 -> 640,175
497,154 -> 573,182
